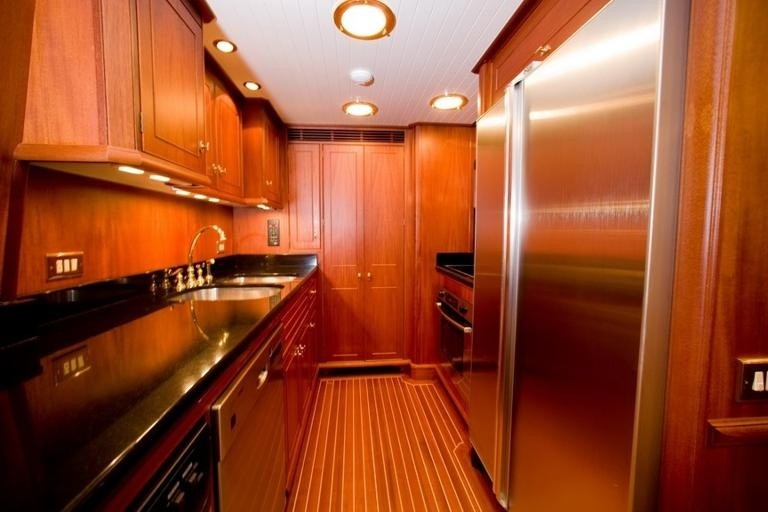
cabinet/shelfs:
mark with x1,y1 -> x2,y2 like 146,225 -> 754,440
288,142 -> 322,250
282,265 -> 319,501
324,142 -> 410,367
131,0 -> 208,176
206,71 -> 285,202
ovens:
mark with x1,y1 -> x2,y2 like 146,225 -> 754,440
428,283 -> 474,426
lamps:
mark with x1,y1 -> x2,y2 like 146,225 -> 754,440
334,0 -> 397,41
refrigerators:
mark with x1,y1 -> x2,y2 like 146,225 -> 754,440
463,1 -> 693,512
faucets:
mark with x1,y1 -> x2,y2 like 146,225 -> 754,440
187,223 -> 228,288
188,303 -> 231,350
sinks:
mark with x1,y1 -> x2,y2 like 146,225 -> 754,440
221,273 -> 299,283
172,285 -> 283,301
